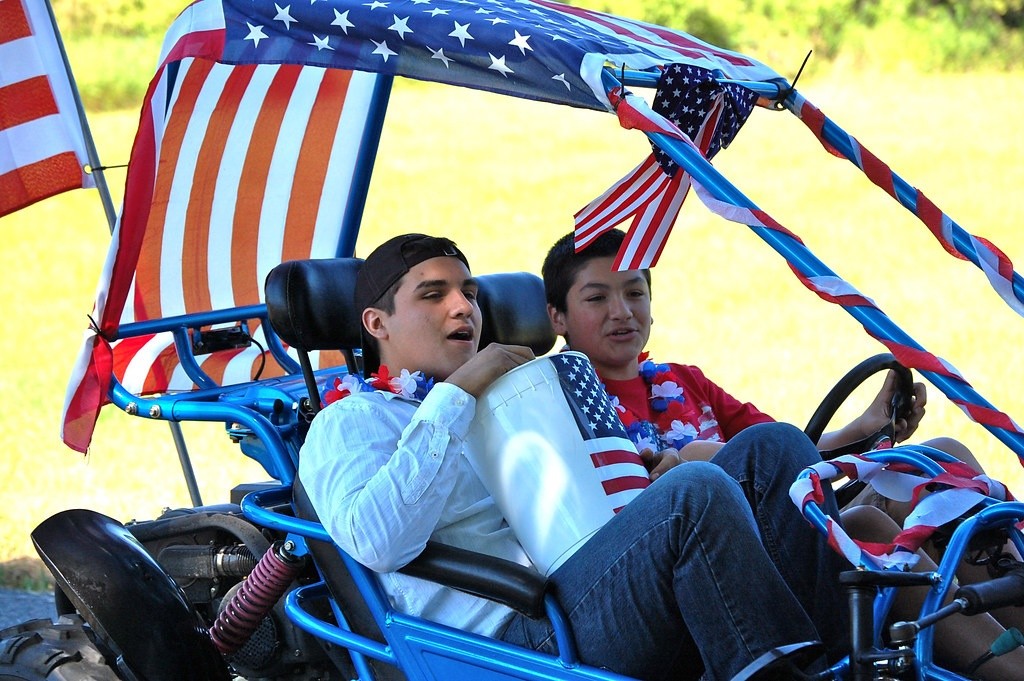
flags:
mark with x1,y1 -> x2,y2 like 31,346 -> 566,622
0,0 -> 97,217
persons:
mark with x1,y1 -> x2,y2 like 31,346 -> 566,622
542,226 -> 1024,681
300,234 -> 869,681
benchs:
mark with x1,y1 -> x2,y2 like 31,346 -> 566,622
265,259 -> 647,681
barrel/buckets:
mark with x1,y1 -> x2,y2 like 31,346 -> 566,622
459,352 -> 654,581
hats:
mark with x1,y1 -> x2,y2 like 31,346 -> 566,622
353,233 -> 471,381
